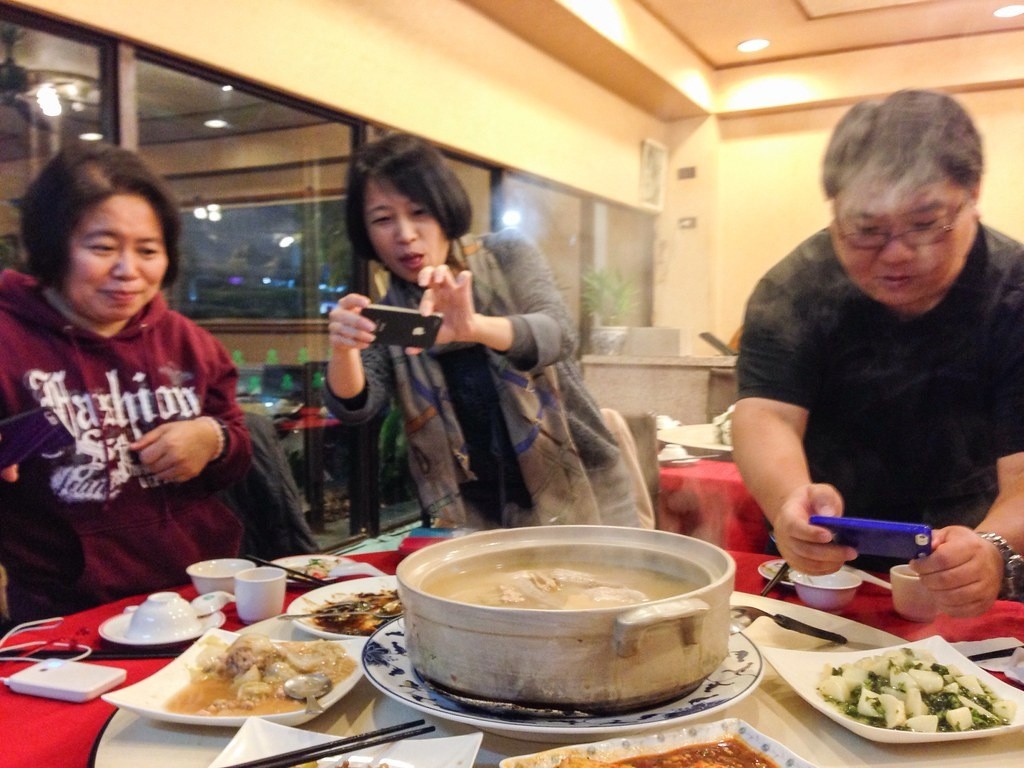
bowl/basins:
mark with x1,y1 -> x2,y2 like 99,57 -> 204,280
185,558 -> 257,598
787,569 -> 862,614
123,592 -> 201,642
655,443 -> 690,462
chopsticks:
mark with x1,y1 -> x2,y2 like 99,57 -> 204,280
244,555 -> 332,588
0,646 -> 187,658
218,718 -> 435,768
661,453 -> 719,462
759,562 -> 789,596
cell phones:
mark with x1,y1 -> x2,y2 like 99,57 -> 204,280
808,516 -> 933,560
354,304 -> 444,349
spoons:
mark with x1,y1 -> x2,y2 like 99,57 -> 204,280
190,592 -> 239,618
330,562 -> 390,578
282,671 -> 333,716
730,603 -> 850,647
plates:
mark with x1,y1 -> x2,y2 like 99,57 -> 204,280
98,606 -> 226,646
205,714 -> 484,768
498,718 -> 816,768
263,554 -> 356,581
758,563 -> 860,586
285,575 -> 417,641
661,456 -> 700,465
100,626 -> 373,727
754,635 -> 1024,743
361,615 -> 762,744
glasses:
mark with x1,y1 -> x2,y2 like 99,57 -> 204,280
834,182 -> 975,249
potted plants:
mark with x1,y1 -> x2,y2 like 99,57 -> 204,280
577,266 -> 642,360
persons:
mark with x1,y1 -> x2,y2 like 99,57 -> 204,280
0,143 -> 253,632
732,88 -> 1024,618
323,133 -> 641,539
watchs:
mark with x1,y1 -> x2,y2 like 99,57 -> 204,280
974,531 -> 1024,601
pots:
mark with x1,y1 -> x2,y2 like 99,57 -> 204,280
392,521 -> 738,717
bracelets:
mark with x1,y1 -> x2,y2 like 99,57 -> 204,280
196,416 -> 225,462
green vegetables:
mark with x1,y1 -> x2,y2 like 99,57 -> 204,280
824,657 -> 1014,733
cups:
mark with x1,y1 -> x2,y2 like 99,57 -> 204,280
888,564 -> 944,623
234,566 -> 286,625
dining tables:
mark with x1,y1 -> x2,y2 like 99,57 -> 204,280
658,459 -> 769,552
0,551 -> 1024,768
